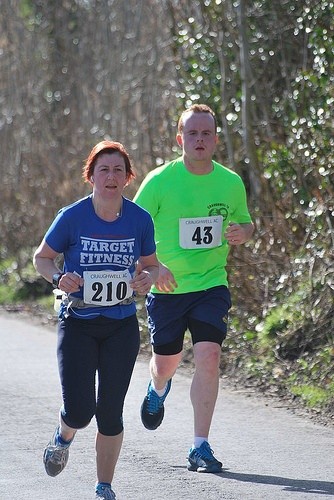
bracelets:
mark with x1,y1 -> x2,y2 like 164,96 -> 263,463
52,270 -> 66,289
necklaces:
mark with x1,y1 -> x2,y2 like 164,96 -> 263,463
93,195 -> 123,218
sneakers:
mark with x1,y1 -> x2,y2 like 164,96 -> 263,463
95,483 -> 116,500
43,425 -> 77,477
187,442 -> 223,473
140,377 -> 172,431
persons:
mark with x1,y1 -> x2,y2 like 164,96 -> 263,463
33,141 -> 158,500
134,104 -> 254,472
